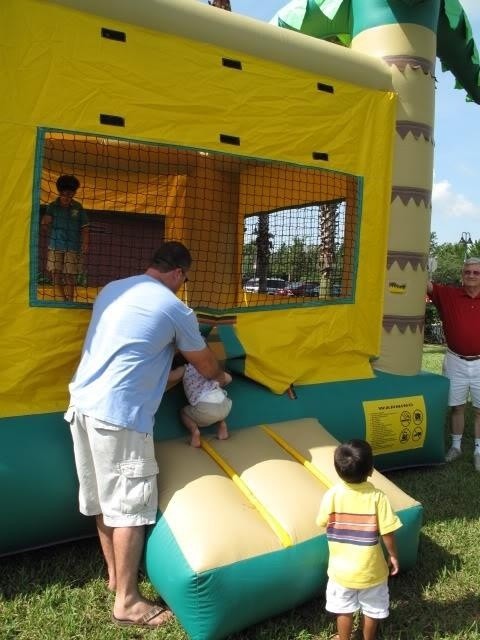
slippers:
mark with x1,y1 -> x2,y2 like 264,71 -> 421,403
112,602 -> 167,629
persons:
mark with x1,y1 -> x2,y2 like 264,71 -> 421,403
425,254 -> 479,474
161,361 -> 236,446
41,176 -> 91,303
64,240 -> 227,625
316,442 -> 403,638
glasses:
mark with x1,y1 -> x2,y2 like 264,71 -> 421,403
464,271 -> 480,275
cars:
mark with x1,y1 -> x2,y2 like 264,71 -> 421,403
243,277 -> 340,297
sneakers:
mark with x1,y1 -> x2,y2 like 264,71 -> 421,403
473,452 -> 480,471
445,447 -> 463,462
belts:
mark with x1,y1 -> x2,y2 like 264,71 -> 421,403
457,355 -> 480,361
